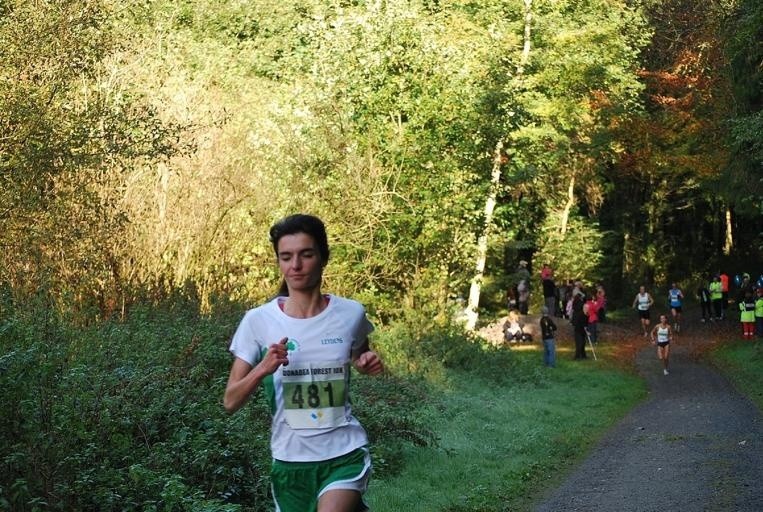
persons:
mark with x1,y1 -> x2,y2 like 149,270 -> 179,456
223,214 -> 384,512
502,260 -> 763,376
